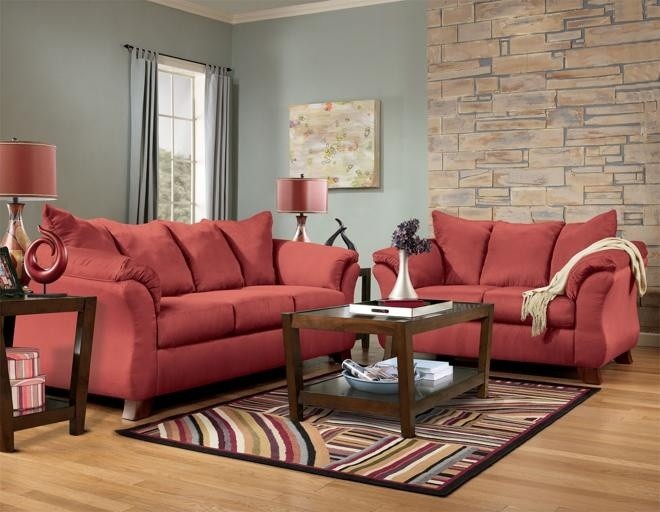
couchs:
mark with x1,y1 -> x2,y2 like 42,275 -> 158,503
372,237 -> 649,385
12,238 -> 361,422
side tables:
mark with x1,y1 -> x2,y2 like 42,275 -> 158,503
360,268 -> 371,350
0,295 -> 97,453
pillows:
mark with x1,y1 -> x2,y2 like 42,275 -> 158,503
42,202 -> 119,255
479,220 -> 566,288
431,210 -> 493,285
549,208 -> 617,296
97,217 -> 195,297
212,211 -> 276,286
161,219 -> 245,292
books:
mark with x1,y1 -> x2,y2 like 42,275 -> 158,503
375,355 -> 454,381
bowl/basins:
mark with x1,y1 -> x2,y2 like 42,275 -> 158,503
342,369 -> 425,394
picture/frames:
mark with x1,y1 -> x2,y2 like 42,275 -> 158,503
0,247 -> 25,298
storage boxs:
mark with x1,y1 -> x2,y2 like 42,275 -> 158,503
10,374 -> 47,418
6,347 -> 41,379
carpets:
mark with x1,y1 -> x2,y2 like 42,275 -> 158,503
114,363 -> 602,497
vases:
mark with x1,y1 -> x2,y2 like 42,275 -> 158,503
388,250 -> 418,301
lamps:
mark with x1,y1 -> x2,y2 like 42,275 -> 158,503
276,174 -> 329,242
0,138 -> 57,294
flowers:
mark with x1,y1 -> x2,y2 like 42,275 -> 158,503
391,218 -> 432,255
